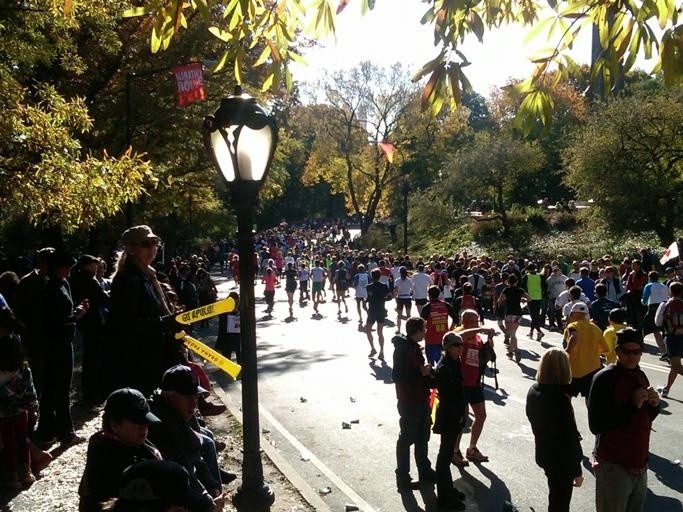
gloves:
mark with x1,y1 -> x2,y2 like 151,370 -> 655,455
163,310 -> 191,334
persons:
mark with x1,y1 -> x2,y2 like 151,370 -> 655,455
393,309 -> 495,499
250,221 -> 370,322
2,220 -> 245,511
366,219 -> 683,396
525,301 -> 608,512
587,328 -> 661,511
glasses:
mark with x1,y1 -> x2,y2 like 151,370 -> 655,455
453,342 -> 463,347
138,239 -> 158,248
620,346 -> 643,356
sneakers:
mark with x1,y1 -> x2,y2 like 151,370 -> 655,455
443,489 -> 467,511
400,470 -> 436,491
199,397 -> 227,416
657,385 -> 669,398
504,338 -> 522,362
466,447 -> 488,461
43,428 -> 85,448
8,449 -> 53,491
220,470 -> 236,484
368,349 -> 384,360
450,450 -> 468,466
660,351 -> 669,361
526,330 -> 544,341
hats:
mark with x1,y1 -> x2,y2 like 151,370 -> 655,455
442,331 -> 463,350
122,224 -> 162,244
665,267 -> 674,274
106,385 -> 164,425
609,308 -> 628,323
569,302 -> 590,316
615,327 -> 643,346
35,247 -> 99,269
161,363 -> 210,398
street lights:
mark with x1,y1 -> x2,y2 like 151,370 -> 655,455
199,82 -> 278,512
